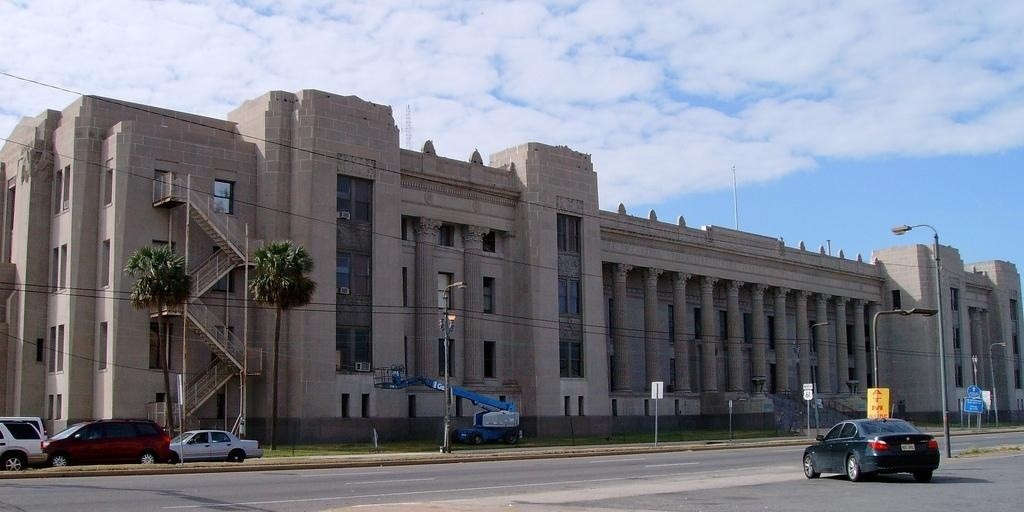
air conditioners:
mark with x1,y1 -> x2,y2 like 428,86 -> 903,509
342,211 -> 350,219
340,287 -> 350,295
355,362 -> 371,372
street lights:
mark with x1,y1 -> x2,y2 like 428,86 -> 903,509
892,223 -> 952,460
438,279 -> 468,451
809,320 -> 831,440
989,342 -> 1007,427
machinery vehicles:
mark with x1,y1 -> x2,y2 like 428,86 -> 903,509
371,364 -> 520,446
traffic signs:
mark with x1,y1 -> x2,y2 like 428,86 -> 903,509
966,384 -> 982,398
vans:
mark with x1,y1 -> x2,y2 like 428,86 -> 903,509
0,415 -> 45,442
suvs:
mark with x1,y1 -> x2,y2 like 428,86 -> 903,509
45,419 -> 171,468
1,423 -> 47,472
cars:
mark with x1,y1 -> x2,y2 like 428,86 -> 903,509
168,430 -> 266,464
802,416 -> 940,484
